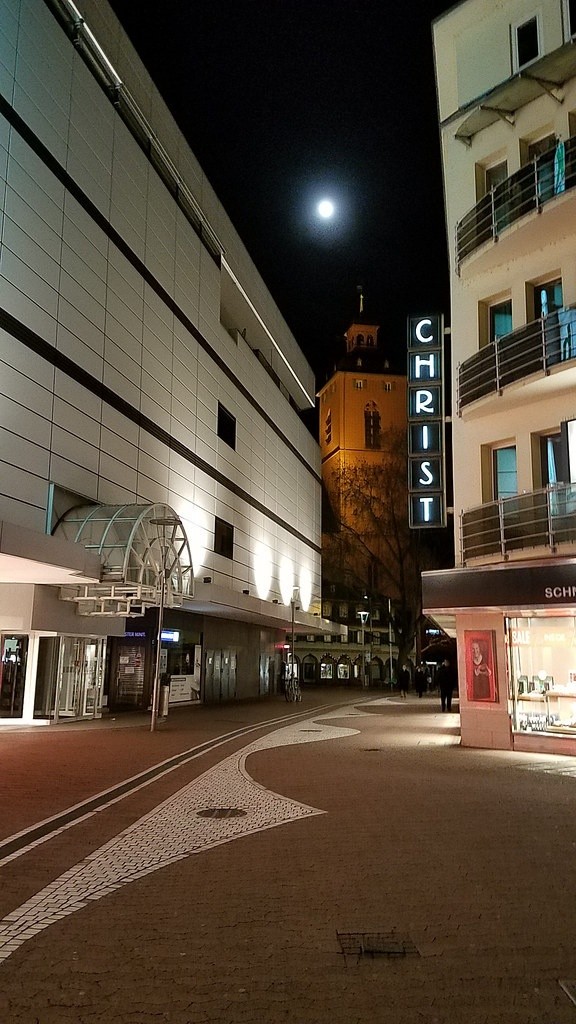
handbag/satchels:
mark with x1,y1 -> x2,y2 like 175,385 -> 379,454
427,677 -> 432,683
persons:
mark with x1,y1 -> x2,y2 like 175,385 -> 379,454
471,641 -> 492,699
396,660 -> 457,712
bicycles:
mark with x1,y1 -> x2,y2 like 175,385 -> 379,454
285,672 -> 302,703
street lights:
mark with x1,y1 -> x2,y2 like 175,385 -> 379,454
286,584 -> 301,678
356,612 -> 370,691
149,516 -> 183,732
